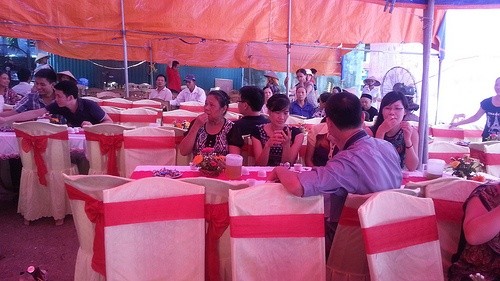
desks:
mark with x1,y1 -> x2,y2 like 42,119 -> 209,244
131,165 -> 500,223
0,127 -> 86,191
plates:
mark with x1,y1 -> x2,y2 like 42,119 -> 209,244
154,173 -> 182,178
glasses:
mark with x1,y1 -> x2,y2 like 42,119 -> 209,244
383,107 -> 404,113
236,99 -> 246,104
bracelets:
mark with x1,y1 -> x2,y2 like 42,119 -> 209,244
406,144 -> 413,149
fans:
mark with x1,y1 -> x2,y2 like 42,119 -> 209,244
380,67 -> 422,112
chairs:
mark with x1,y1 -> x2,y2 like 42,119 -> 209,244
10,83 -> 500,281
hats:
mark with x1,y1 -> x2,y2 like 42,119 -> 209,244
56,70 -> 78,84
264,71 -> 280,80
36,53 -> 51,62
184,74 -> 196,82
362,76 -> 382,87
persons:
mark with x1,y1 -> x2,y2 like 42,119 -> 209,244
34,53 -> 51,77
364,91 -> 419,171
167,74 -> 206,107
227,86 -> 271,156
180,90 -> 235,158
148,73 -> 173,112
56,71 -> 78,84
268,93 -> 404,251
0,69 -> 67,126
0,67 -> 34,112
166,60 -> 183,93
449,77 -> 500,142
0,81 -> 114,130
262,68 -> 419,124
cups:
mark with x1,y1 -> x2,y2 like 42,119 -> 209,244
226,154 -> 243,177
294,164 -> 302,171
258,169 -> 266,177
425,160 -> 445,180
243,168 -> 249,175
67,127 -> 79,133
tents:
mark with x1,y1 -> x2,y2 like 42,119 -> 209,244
0,0 -> 500,80
